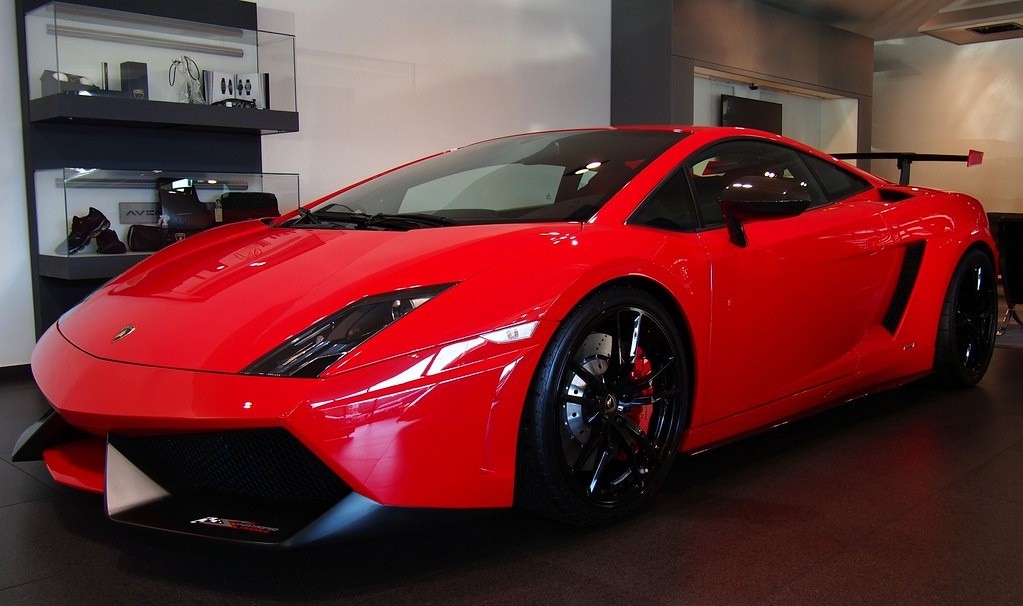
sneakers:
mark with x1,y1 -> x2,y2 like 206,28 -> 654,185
96,229 -> 126,255
55,207 -> 110,255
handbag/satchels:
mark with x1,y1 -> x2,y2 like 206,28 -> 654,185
215,191 -> 281,227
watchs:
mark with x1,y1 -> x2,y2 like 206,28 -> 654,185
221,78 -> 251,96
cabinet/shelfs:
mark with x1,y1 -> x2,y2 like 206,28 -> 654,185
15,0 -> 300,343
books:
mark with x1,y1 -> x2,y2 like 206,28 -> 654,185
202,70 -> 271,110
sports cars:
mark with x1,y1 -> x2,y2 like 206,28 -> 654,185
7,125 -> 1001,553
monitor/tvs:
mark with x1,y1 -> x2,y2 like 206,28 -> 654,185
720,93 -> 782,137
